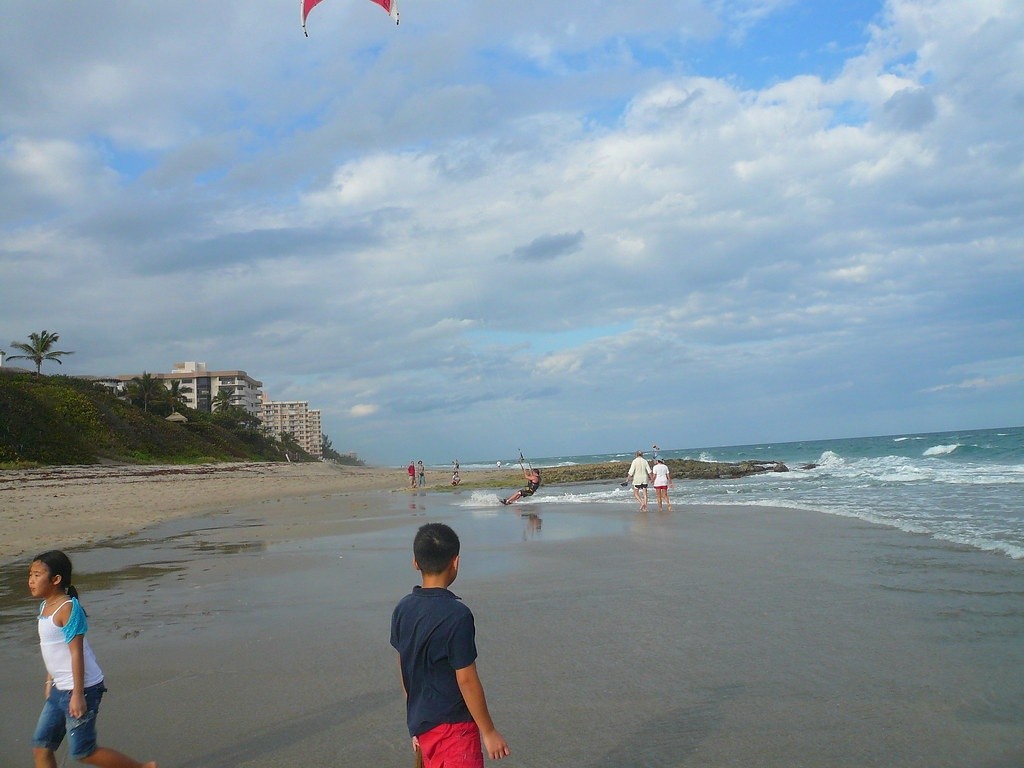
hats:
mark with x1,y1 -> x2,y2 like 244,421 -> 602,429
653,454 -> 664,463
617,480 -> 631,487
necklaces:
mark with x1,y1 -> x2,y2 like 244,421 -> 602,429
43,595 -> 70,608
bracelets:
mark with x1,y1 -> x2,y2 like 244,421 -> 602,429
44,681 -> 53,684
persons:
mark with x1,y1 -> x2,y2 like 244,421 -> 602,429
389,523 -> 509,768
451,459 -> 461,486
29,550 -> 156,768
626,445 -> 673,512
408,461 -> 425,489
497,460 -> 541,506
